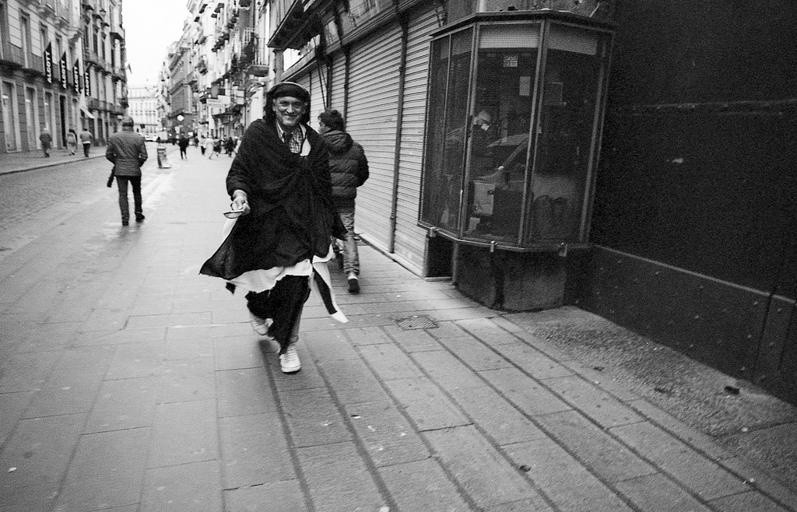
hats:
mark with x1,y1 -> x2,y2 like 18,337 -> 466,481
265,82 -> 311,125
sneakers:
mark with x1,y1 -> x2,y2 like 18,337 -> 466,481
135,215 -> 144,222
249,312 -> 268,336
277,344 -> 301,373
346,271 -> 360,293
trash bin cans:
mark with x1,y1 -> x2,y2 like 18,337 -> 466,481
158,147 -> 166,167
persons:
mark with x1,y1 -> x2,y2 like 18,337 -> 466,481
136,127 -> 144,141
168,126 -> 242,160
80,128 -> 93,157
105,117 -> 149,226
39,127 -> 53,157
198,82 -> 350,376
443,108 -> 496,232
316,110 -> 370,294
66,128 -> 77,156
